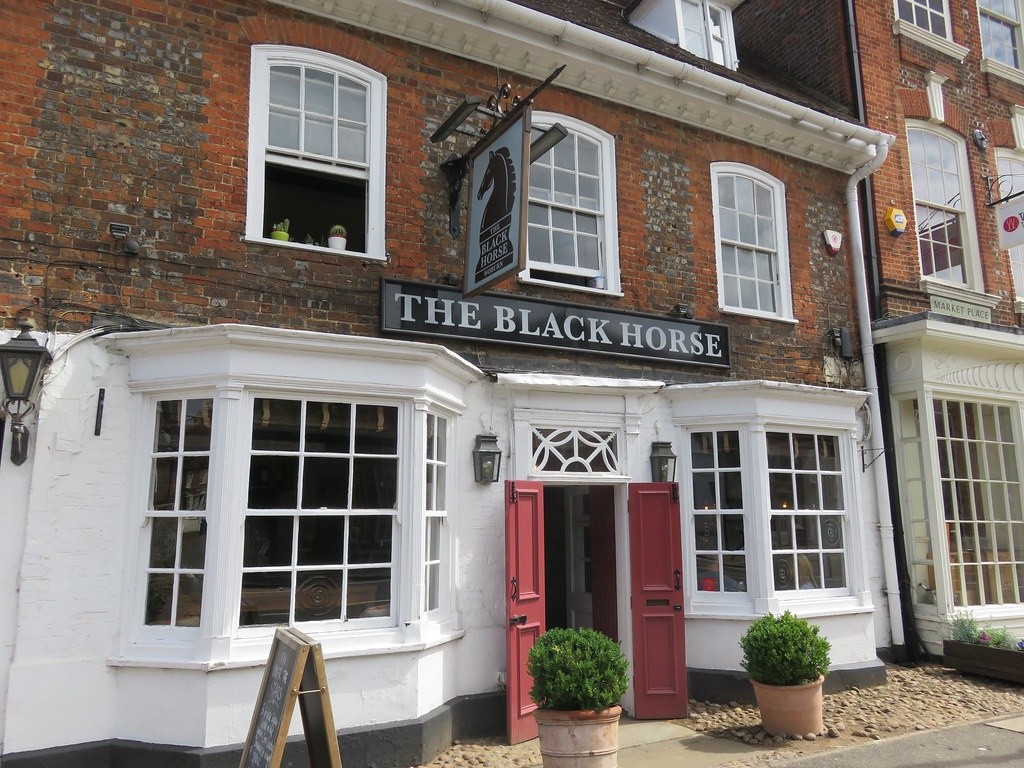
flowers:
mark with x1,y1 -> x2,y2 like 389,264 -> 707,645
949,609 -> 1024,651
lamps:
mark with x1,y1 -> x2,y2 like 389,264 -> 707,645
0,317 -> 54,467
649,440 -> 678,482
472,434 -> 503,485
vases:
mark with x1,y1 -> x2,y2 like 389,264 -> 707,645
943,640 -> 1024,683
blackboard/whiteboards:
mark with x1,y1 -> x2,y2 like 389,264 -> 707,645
237,628 -> 307,768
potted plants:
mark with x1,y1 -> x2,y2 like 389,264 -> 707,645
525,626 -> 631,768
737,608 -> 832,737
327,225 -> 348,250
271,219 -> 289,241
